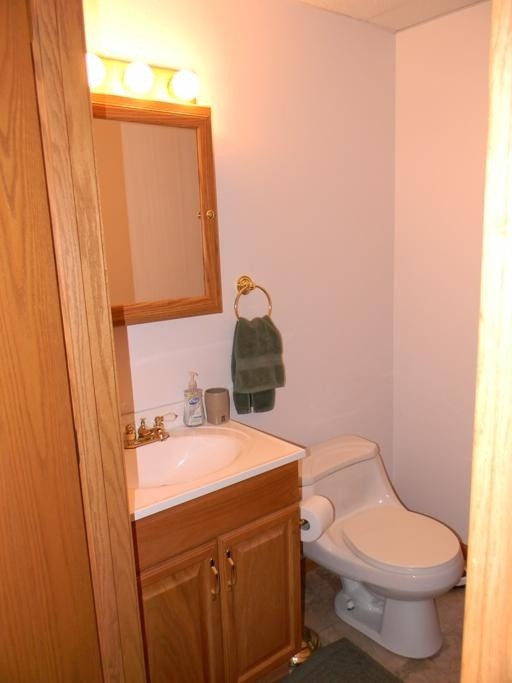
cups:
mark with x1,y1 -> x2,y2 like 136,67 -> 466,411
205,387 -> 230,425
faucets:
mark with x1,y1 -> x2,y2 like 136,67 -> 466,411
138,415 -> 165,442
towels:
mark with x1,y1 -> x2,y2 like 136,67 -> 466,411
231,317 -> 286,414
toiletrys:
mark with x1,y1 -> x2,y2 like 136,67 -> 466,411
183,370 -> 206,426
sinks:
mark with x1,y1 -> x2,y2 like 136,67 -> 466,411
124,427 -> 250,484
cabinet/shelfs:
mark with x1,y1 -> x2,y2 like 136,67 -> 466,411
1,0 -> 223,682
137,464 -> 304,680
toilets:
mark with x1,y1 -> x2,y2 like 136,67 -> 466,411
297,432 -> 465,660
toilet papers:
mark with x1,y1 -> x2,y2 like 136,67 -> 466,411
299,495 -> 335,543
